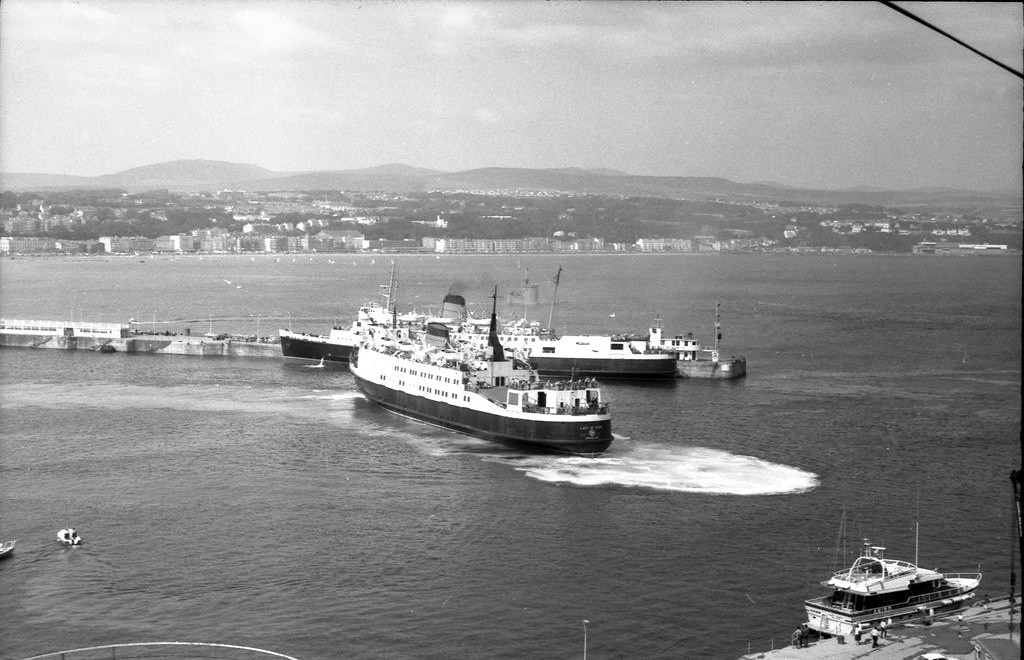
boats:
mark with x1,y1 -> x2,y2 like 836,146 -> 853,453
57,528 -> 82,545
801,485 -> 982,637
278,251 -> 747,379
0,539 -> 16,557
350,285 -> 614,459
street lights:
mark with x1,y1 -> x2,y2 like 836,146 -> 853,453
154,311 -> 157,337
258,314 -> 261,339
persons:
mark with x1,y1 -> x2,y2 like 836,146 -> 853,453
880,619 -> 887,638
854,625 -> 861,645
793,626 -> 802,648
958,614 -> 964,624
802,625 -> 809,647
64,528 -> 78,538
870,627 -> 880,648
